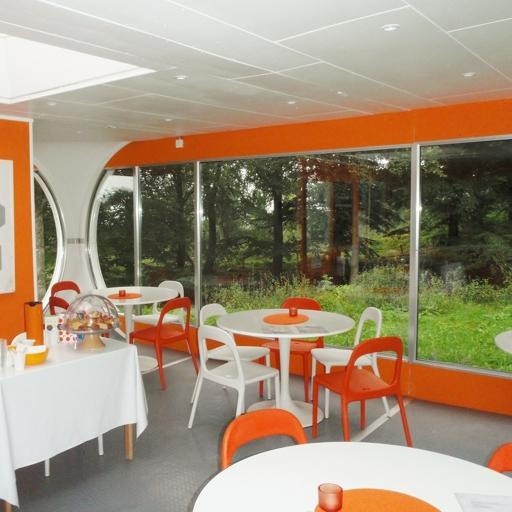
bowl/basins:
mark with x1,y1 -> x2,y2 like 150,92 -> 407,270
22,345 -> 49,366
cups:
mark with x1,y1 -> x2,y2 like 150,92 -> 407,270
119,289 -> 125,297
289,307 -> 297,317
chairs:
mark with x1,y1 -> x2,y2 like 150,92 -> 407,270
311,335 -> 411,448
257,297 -> 328,406
221,410 -> 308,471
488,443 -> 512,473
132,282 -> 192,370
50,296 -> 70,316
184,324 -> 280,430
310,306 -> 395,420
51,282 -> 80,299
128,296 -> 201,390
198,302 -> 273,402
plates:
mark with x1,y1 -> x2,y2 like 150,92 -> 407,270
264,313 -> 309,326
108,293 -> 143,299
313,487 -> 442,512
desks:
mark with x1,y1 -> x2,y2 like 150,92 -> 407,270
193,442 -> 512,512
89,287 -> 179,371
0,336 -> 149,512
495,331 -> 512,354
215,308 -> 355,428
